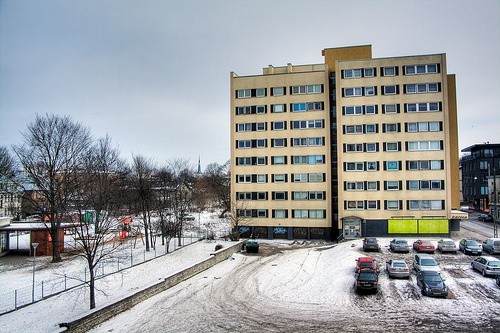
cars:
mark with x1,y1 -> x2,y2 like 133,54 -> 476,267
470,255 -> 500,278
477,213 -> 493,222
176,211 -> 195,221
437,238 -> 457,254
389,238 -> 410,254
385,258 -> 410,279
482,237 -> 500,255
353,269 -> 380,295
362,237 -> 379,252
458,238 -> 484,256
412,254 -> 441,275
354,256 -> 376,274
416,270 -> 448,297
413,239 -> 436,254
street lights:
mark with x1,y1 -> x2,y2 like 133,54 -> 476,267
31,242 -> 39,303
